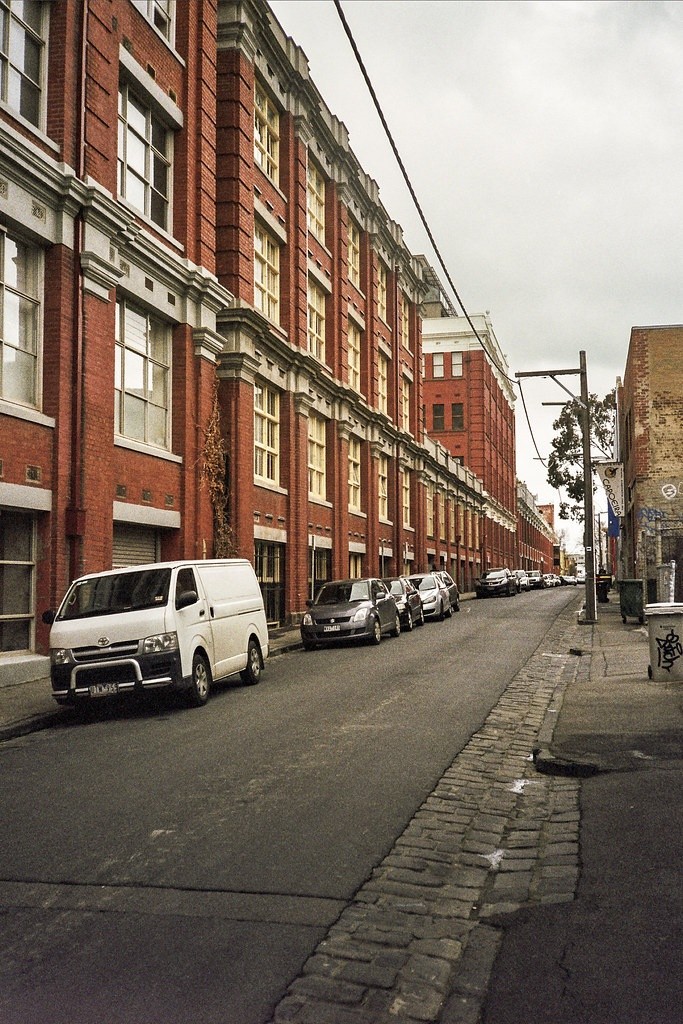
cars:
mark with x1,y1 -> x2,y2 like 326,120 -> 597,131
404,572 -> 453,622
377,577 -> 423,633
425,570 -> 462,611
301,578 -> 402,648
511,568 -> 585,593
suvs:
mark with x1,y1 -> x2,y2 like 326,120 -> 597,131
473,567 -> 517,600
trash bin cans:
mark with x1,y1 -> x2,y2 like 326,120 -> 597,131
596,580 -> 609,603
617,578 -> 657,624
643,602 -> 683,682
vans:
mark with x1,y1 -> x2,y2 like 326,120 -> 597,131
42,558 -> 270,718
